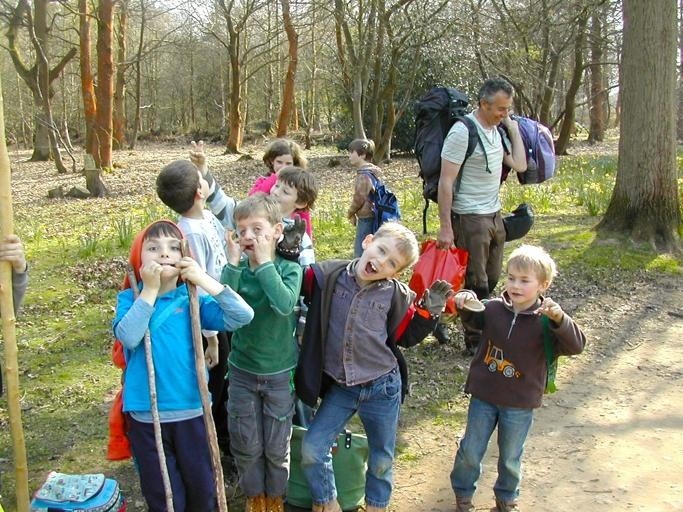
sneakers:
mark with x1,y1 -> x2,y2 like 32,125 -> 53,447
456,496 -> 473,512
312,499 -> 387,512
497,497 -> 519,512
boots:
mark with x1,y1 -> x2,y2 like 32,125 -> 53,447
245,494 -> 283,512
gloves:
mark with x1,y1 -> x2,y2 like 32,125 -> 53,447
279,214 -> 306,250
421,280 -> 454,315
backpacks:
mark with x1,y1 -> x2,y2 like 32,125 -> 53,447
505,114 -> 555,185
414,88 -> 504,203
29,471 -> 127,512
356,170 -> 401,234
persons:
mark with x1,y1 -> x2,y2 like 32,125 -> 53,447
0,234 -> 28,312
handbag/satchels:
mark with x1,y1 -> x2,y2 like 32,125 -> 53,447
502,204 -> 535,242
286,424 -> 373,509
411,239 -> 468,315
